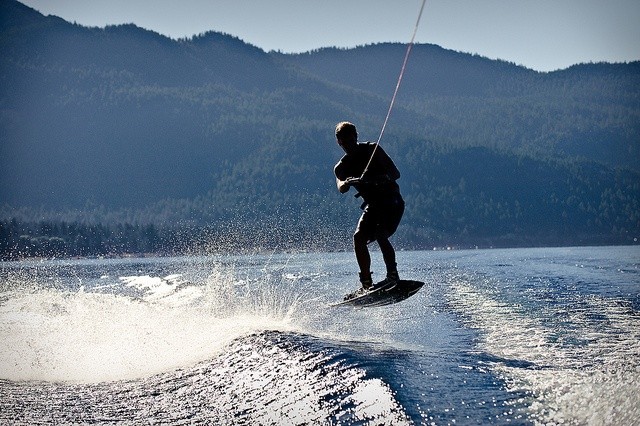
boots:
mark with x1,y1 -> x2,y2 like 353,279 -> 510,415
378,263 -> 400,284
354,272 -> 373,297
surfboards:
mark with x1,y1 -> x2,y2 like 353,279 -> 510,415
327,279 -> 424,311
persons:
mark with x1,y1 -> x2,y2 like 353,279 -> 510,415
333,121 -> 405,292
